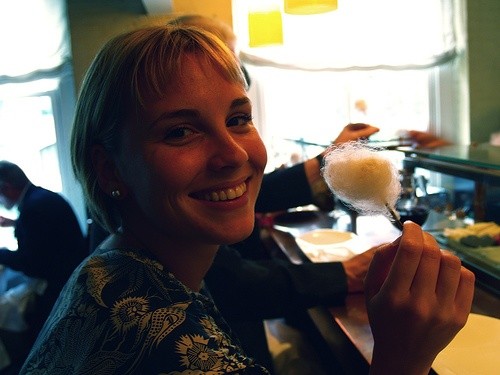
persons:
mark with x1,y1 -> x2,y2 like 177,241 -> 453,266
18,23 -> 474,375
168,14 -> 395,369
0,162 -> 85,375
